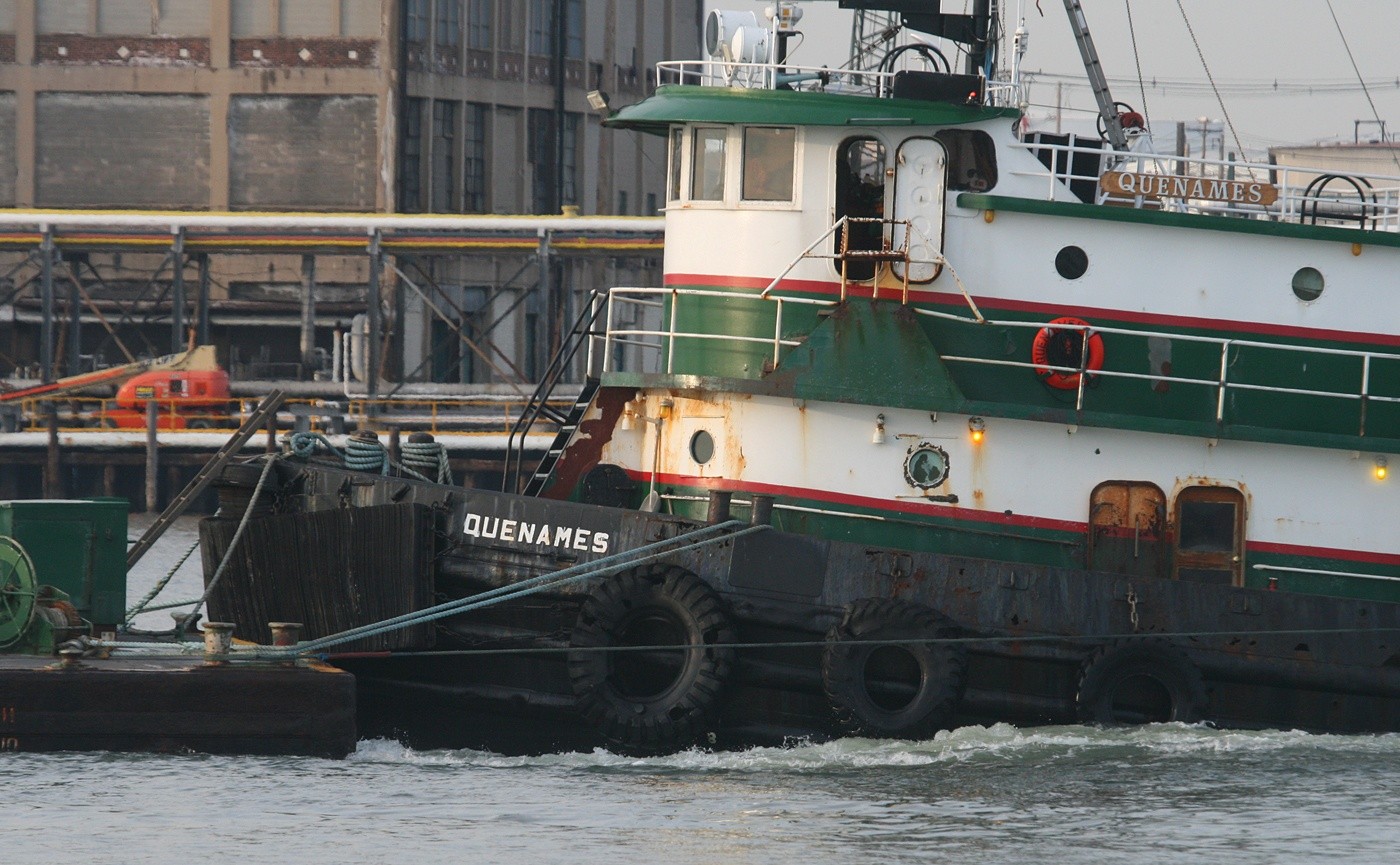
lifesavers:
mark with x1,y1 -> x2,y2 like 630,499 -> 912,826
819,596 -> 966,737
1062,632 -> 1201,729
1032,317 -> 1104,389
566,562 -> 739,751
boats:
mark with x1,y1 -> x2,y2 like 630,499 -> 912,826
1,0 -> 1400,761
